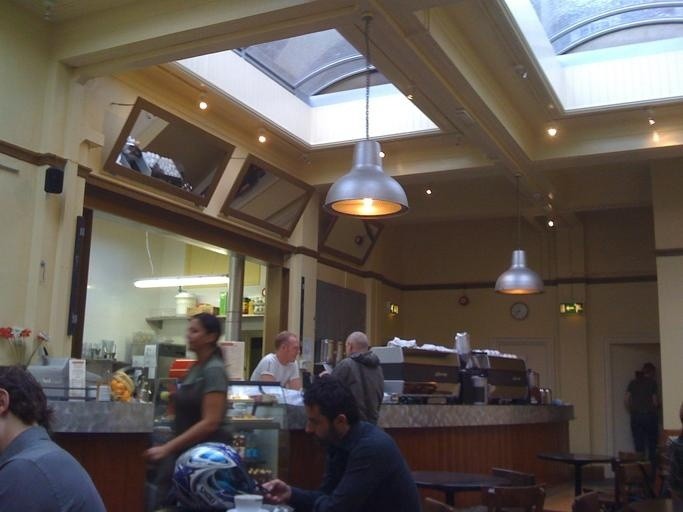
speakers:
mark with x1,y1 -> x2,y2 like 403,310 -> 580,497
45,168 -> 63,193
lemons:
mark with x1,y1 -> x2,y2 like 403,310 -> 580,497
111,380 -> 130,400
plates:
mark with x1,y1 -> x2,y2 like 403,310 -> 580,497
226,507 -> 270,512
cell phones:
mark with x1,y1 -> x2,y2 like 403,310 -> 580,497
256,484 -> 271,494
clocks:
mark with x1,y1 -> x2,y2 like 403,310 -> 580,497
510,302 -> 529,321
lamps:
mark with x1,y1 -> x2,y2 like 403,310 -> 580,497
133,230 -> 233,289
322,8 -> 411,221
493,173 -> 545,296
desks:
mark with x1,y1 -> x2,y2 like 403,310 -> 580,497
157,452 -> 614,511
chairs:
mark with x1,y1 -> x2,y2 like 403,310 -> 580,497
424,445 -> 682,511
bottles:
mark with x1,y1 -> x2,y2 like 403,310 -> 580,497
81,339 -> 118,360
229,430 -> 262,459
242,297 -> 265,315
525,369 -> 540,404
539,387 -> 551,405
320,338 -> 345,363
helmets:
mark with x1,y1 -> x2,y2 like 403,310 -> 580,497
170,441 -> 256,510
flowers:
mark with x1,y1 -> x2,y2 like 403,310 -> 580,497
0,325 -> 49,370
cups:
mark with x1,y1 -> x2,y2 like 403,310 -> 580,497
234,494 -> 264,510
134,378 -> 150,402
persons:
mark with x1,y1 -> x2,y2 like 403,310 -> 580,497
318,330 -> 385,496
624,361 -> 661,478
260,372 -> 419,511
249,329 -> 302,391
659,403 -> 683,500
0,365 -> 110,511
140,312 -> 228,511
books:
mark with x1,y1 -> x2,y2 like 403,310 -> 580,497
320,364 -> 334,379
321,336 -> 348,365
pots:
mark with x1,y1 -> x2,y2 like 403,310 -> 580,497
175,292 -> 197,315
